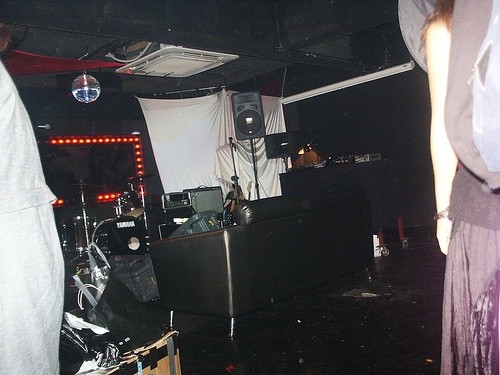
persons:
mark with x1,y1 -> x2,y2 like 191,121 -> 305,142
0,56 -> 65,375
416,0 -> 500,375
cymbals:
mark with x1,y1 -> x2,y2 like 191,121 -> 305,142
127,173 -> 158,181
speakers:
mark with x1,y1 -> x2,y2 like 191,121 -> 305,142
264,131 -> 299,159
231,91 -> 266,140
183,186 -> 224,214
169,209 -> 221,238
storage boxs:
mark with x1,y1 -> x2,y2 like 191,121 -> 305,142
108,254 -> 160,303
157,187 -> 223,241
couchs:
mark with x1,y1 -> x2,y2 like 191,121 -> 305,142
148,193 -> 376,318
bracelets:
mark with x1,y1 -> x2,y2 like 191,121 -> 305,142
433,208 -> 450,220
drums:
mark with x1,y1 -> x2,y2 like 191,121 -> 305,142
112,192 -> 144,218
62,216 -> 100,252
92,217 -> 115,261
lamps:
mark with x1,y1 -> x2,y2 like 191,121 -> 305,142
278,58 -> 417,105
71,49 -> 101,104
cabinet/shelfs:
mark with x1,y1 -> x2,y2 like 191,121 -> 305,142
279,161 -> 417,256
